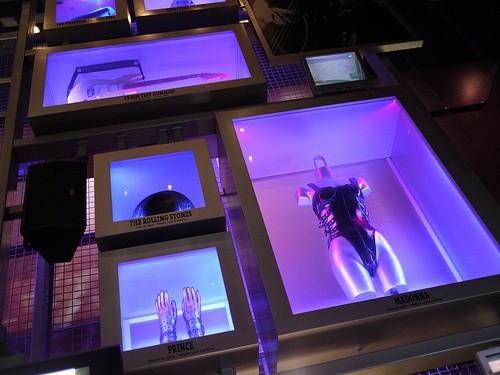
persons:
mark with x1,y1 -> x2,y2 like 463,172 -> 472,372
295,166 -> 409,301
154,286 -> 205,344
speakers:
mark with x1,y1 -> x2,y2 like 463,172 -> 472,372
20,156 -> 87,263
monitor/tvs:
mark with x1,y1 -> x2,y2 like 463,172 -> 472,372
299,45 -> 385,97
